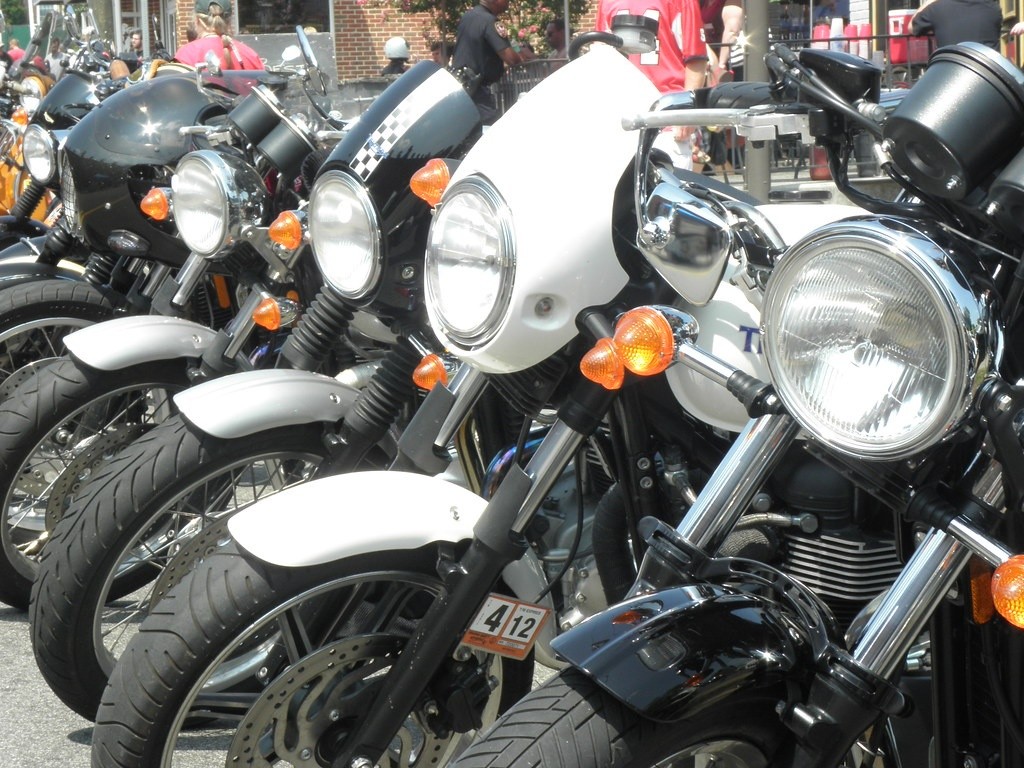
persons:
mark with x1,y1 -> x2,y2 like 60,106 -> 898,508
133,30 -> 144,57
187,28 -> 198,43
30,50 -> 45,70
448,0 -> 520,127
909,0 -> 1004,56
45,39 -> 70,81
430,42 -> 454,66
700,0 -> 779,176
381,37 -> 411,78
175,0 -> 265,73
154,42 -> 172,60
595,0 -> 709,221
6,39 -> 25,61
519,18 -> 585,77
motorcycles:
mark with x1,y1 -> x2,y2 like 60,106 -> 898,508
0,33 -> 1024,768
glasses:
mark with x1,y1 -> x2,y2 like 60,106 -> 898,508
544,31 -> 554,37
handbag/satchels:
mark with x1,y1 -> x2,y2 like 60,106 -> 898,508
710,129 -> 727,165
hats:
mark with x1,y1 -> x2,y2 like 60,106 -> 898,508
0,33 -> 5,47
195,0 -> 232,18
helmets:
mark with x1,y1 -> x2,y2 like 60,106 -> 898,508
384,37 -> 410,60
